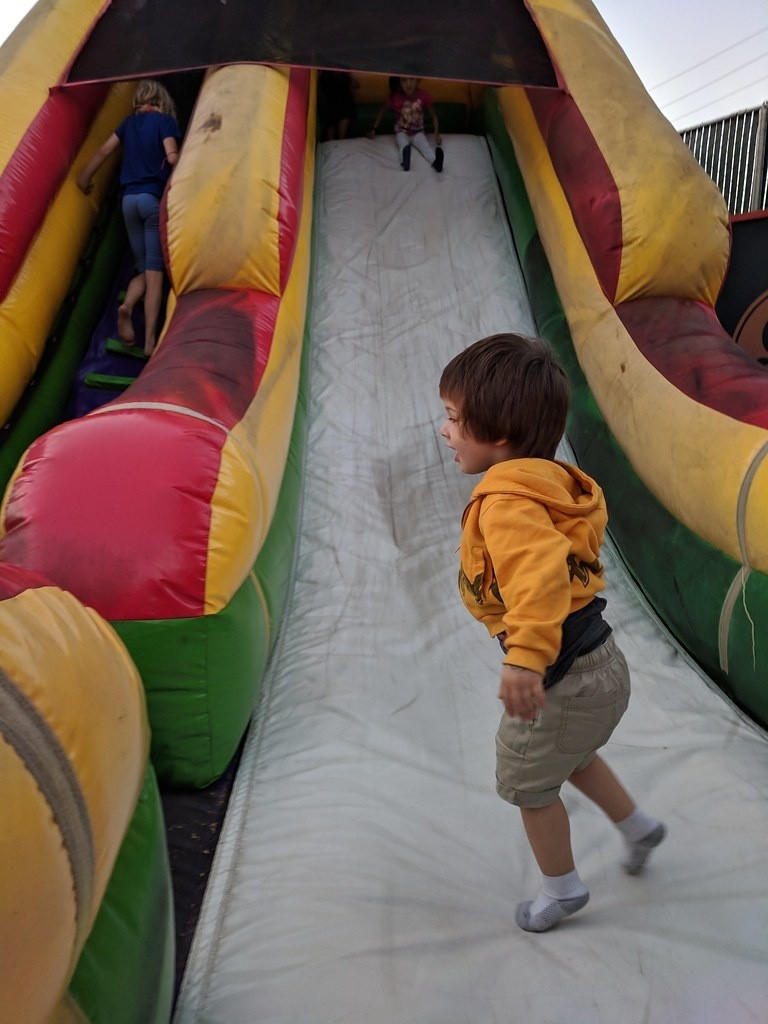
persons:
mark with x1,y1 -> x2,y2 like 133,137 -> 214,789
437,333 -> 669,933
366,74 -> 444,173
77,79 -> 182,357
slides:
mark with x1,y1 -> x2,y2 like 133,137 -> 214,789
0,1 -> 767,1024
